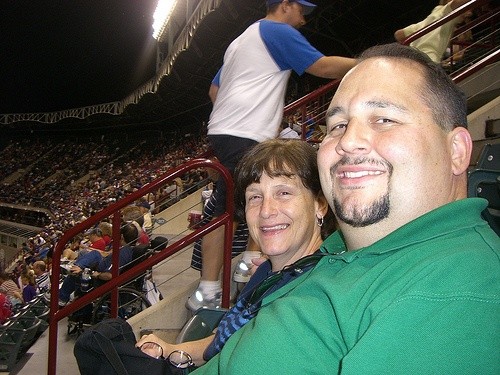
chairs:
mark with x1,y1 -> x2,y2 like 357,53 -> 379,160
0,297 -> 50,372
467,142 -> 500,235
176,305 -> 228,345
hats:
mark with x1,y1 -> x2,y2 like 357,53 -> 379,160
135,201 -> 151,209
266,0 -> 317,15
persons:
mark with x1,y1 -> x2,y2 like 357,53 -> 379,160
186,43 -> 500,375
186,0 -> 361,315
0,20 -> 472,375
135,137 -> 326,375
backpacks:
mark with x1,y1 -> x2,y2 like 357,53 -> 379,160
74,318 -> 183,375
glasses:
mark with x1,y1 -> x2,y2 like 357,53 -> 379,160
136,342 -> 196,372
246,254 -> 328,315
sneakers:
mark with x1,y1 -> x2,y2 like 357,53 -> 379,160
234,259 -> 252,282
44,294 -> 66,306
186,287 -> 232,310
